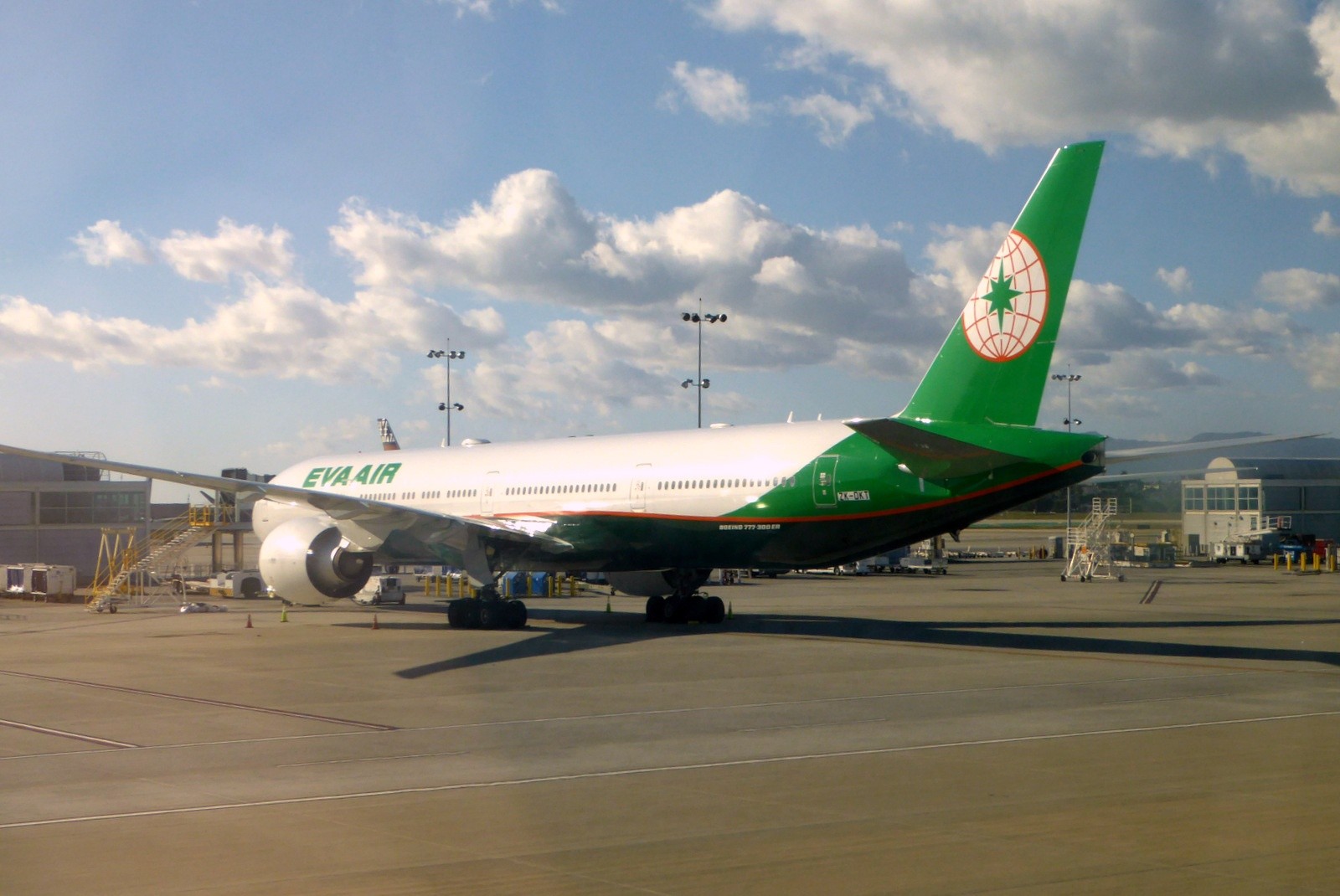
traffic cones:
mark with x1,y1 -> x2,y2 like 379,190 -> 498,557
370,612 -> 379,630
280,606 -> 289,622
244,613 -> 255,628
603,602 -> 613,613
725,608 -> 735,620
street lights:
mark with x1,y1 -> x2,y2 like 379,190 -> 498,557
681,296 -> 728,428
426,338 -> 466,446
1051,363 -> 1084,559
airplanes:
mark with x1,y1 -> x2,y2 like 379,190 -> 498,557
0,138 -> 1107,632
